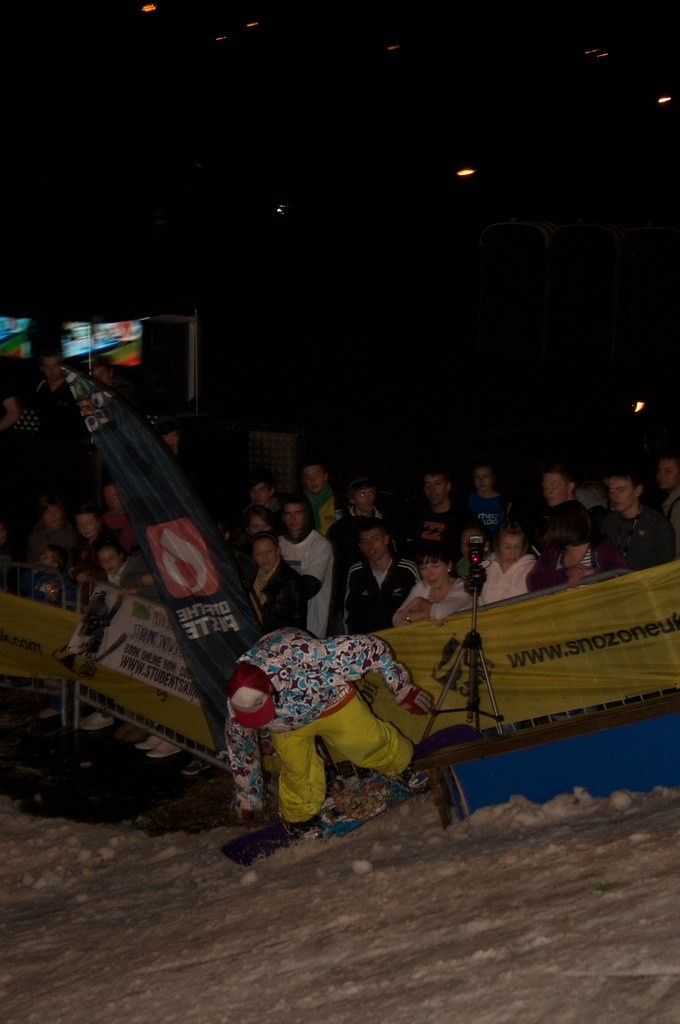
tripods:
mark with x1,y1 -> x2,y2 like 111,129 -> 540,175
420,584 -> 503,745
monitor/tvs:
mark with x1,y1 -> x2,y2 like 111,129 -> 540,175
58,317 -> 151,375
0,312 -> 39,363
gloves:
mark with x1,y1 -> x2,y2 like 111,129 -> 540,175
234,808 -> 265,825
397,687 -> 434,716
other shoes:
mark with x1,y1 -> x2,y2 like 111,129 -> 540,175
79,711 -> 114,730
147,740 -> 181,758
181,758 -> 211,775
124,723 -> 155,742
134,740 -> 159,750
112,716 -> 143,739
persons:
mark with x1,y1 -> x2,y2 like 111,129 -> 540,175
468,465 -> 504,538
247,531 -> 307,634
344,521 -> 420,636
225,626 -> 434,839
526,498 -> 630,593
0,349 -> 680,773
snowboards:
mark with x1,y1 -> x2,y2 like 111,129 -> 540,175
221,723 -> 485,868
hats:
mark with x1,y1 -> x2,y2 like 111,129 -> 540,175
226,664 -> 276,729
